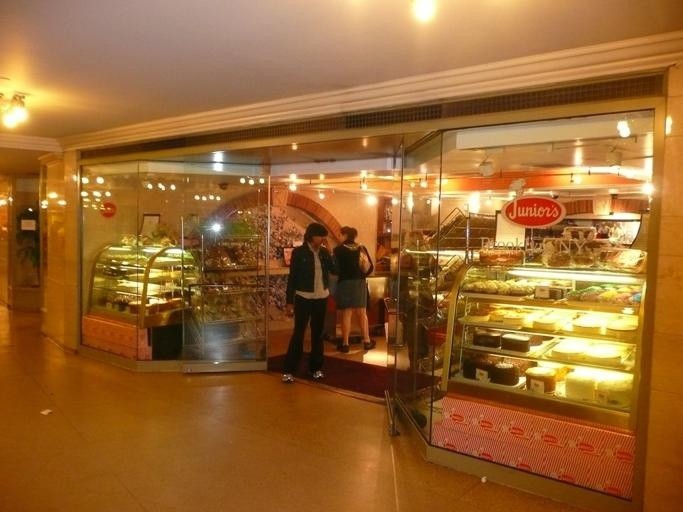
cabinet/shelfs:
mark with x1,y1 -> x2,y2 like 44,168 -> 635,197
441,263 -> 646,433
183,230 -> 268,351
81,239 -> 202,358
414,206 -> 497,405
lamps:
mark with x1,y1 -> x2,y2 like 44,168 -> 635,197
0,78 -> 28,130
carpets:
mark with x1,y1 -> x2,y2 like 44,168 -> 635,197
265,350 -> 443,407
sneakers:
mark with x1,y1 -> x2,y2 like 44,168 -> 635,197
364,338 -> 376,350
312,371 -> 324,380
336,341 -> 350,353
281,372 -> 295,384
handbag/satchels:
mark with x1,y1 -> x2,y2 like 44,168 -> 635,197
359,251 -> 371,274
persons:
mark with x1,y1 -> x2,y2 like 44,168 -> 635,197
280,223 -> 331,383
329,225 -> 375,352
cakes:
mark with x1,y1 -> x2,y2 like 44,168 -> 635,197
479,249 -> 524,266
542,226 -> 612,266
461,278 -> 643,410
